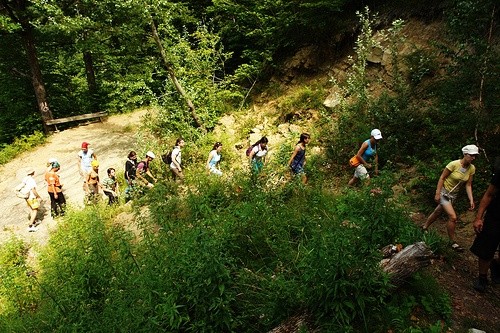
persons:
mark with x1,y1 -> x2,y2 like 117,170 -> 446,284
132,151 -> 157,189
104,168 -> 116,205
348,129 -> 383,187
78,142 -> 97,190
422,145 -> 480,252
87,161 -> 99,195
249,137 -> 269,173
125,151 -> 138,202
170,138 -> 184,185
288,133 -> 310,183
46,161 -> 66,218
24,170 -> 46,232
206,142 -> 225,176
469,168 -> 500,294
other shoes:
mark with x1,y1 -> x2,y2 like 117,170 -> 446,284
449,241 -> 464,252
472,279 -> 488,292
27,225 -> 37,232
421,227 -> 429,235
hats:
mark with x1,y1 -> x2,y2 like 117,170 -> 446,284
146,151 -> 156,159
461,144 -> 480,155
370,129 -> 383,140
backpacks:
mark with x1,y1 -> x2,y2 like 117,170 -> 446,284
161,152 -> 172,165
246,146 -> 259,156
128,161 -> 146,177
15,179 -> 31,200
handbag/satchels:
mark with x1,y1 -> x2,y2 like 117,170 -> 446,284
349,155 -> 360,167
